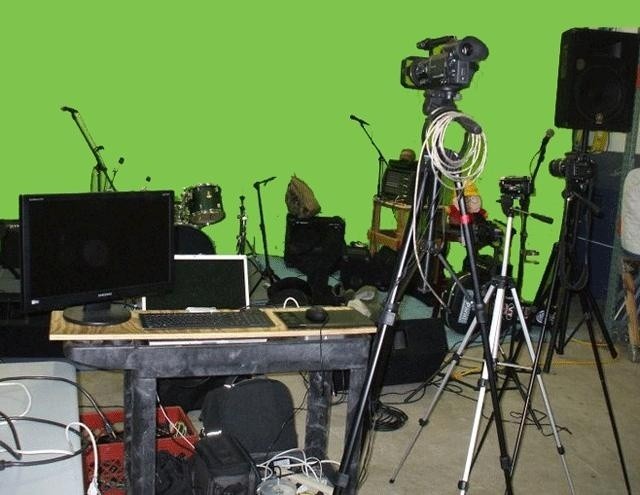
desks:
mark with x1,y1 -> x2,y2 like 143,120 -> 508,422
45,301 -> 382,495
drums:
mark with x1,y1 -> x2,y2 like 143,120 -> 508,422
173,200 -> 202,231
180,182 -> 226,226
173,222 -> 216,254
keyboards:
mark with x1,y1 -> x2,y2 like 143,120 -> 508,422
139,311 -> 276,328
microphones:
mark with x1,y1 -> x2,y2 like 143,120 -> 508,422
542,128 -> 554,145
61,106 -> 79,113
350,114 -> 370,126
240,195 -> 245,205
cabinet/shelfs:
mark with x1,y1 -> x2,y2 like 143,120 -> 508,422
365,193 -> 445,294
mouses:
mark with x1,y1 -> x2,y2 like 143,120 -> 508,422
306,306 -> 327,323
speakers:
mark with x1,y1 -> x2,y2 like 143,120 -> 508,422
554,27 -> 640,133
332,318 -> 447,391
284,214 -> 345,269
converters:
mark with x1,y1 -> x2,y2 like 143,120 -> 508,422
269,459 -> 292,475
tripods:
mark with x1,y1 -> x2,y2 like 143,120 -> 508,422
390,215 -> 576,495
249,191 -> 282,299
515,183 -> 617,374
332,149 -> 514,495
471,214 -> 632,495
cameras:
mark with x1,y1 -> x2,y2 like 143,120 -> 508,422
549,151 -> 593,180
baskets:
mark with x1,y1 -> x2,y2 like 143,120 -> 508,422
79,405 -> 197,494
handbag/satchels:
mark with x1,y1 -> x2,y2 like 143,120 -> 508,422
156,377 -> 298,495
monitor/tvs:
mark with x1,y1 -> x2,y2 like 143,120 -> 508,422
141,255 -> 250,312
19,189 -> 174,327
